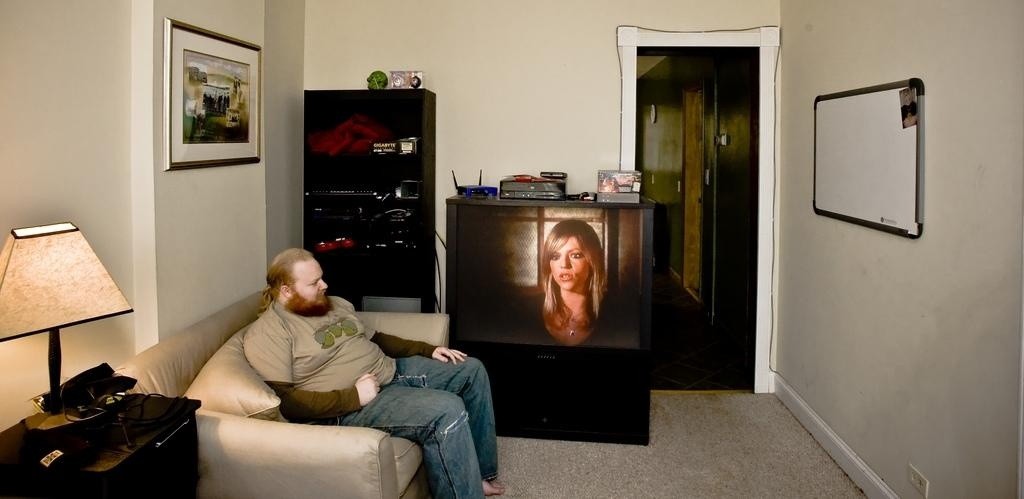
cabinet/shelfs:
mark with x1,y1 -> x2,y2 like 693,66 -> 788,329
304,88 -> 436,313
0,393 -> 202,499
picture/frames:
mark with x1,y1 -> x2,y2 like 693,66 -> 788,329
162,17 -> 262,172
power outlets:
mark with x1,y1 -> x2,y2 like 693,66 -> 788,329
907,462 -> 929,499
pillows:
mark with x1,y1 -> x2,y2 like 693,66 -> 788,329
183,320 -> 281,421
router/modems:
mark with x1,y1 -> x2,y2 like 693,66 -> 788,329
452,169 -> 497,198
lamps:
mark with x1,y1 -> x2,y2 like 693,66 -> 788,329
0,221 -> 133,431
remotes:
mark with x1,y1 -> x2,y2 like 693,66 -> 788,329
541,172 -> 567,179
583,192 -> 596,201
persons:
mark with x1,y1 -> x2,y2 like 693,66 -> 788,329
542,219 -> 609,341
245,246 -> 505,498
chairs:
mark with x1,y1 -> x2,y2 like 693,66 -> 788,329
114,286 -> 450,499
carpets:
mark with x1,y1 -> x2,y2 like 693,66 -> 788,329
428,392 -> 868,499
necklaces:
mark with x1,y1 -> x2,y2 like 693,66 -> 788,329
563,322 -> 576,337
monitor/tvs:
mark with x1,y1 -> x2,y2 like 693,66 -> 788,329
446,193 -> 655,360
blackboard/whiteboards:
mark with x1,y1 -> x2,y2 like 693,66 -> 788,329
813,77 -> 925,239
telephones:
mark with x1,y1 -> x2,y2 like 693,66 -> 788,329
60,363 -> 137,408
